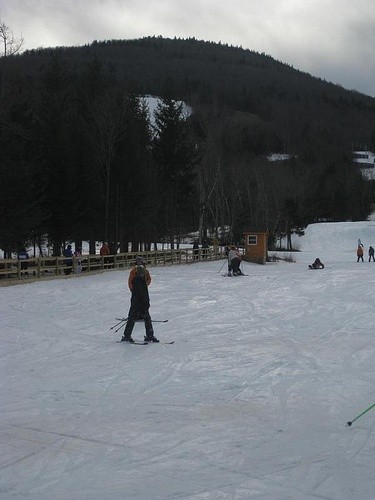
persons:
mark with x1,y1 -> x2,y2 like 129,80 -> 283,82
309,258 -> 324,269
229,246 -> 240,276
357,246 -> 364,262
63,245 -> 72,275
100,241 -> 109,269
72,247 -> 82,276
129,257 -> 151,292
358,238 -> 364,247
226,246 -> 244,276
368,246 -> 375,262
121,266 -> 159,342
108,243 -> 117,268
19,248 -> 28,276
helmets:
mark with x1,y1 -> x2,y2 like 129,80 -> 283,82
231,245 -> 236,250
136,257 -> 143,264
227,247 -> 232,251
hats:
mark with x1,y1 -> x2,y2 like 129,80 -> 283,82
136,266 -> 145,273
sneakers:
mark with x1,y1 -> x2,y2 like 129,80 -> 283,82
144,333 -> 159,343
121,336 -> 135,343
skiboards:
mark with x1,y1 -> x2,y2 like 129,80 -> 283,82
115,317 -> 169,323
221,274 -> 249,277
116,340 -> 174,345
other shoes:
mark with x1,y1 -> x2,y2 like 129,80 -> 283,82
357,259 -> 375,262
228,271 -> 242,276
